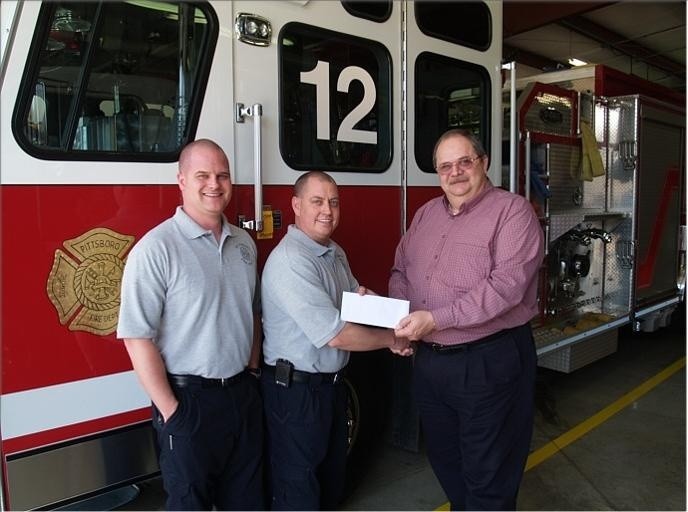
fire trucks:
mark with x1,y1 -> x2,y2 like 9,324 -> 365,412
0,0 -> 686,512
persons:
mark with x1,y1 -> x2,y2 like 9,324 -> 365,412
389,130 -> 544,511
116,139 -> 261,510
260,170 -> 414,511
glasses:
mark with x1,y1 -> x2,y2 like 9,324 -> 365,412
435,154 -> 485,171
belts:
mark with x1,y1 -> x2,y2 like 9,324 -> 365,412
169,370 -> 245,389
419,335 -> 497,354
264,364 -> 344,384
